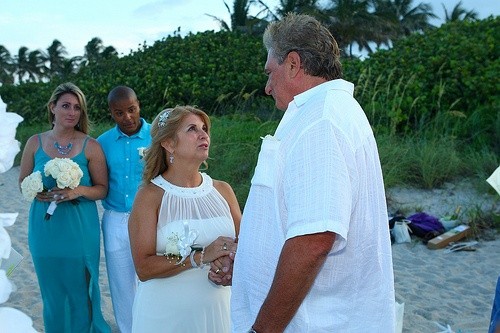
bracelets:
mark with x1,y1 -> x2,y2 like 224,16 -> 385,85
250,326 -> 257,333
200,247 -> 205,268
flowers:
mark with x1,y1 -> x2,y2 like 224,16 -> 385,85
43,157 -> 84,221
157,111 -> 170,126
163,221 -> 200,267
21,171 -> 82,207
137,147 -> 147,159
260,134 -> 281,140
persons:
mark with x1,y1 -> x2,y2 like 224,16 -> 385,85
18,82 -> 111,333
96,86 -> 155,333
228,19 -> 399,333
126,106 -> 242,333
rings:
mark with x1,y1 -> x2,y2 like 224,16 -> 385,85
224,243 -> 228,250
215,269 -> 220,273
61,195 -> 64,199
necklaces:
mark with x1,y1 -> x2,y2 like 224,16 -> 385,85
51,130 -> 75,155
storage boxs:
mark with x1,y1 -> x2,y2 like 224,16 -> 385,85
428,225 -> 471,250
